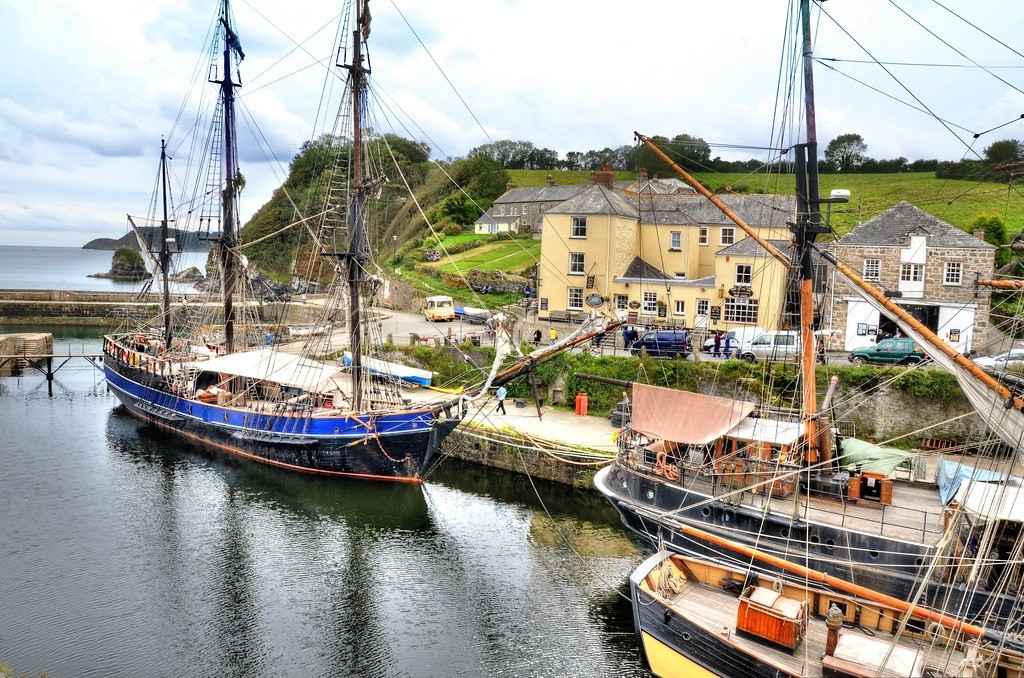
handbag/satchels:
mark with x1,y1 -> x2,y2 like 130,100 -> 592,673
555,330 -> 558,339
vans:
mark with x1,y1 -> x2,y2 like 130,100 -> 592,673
737,329 -> 843,364
631,330 -> 693,360
703,327 -> 764,357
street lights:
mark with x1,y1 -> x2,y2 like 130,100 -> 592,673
393,236 -> 397,273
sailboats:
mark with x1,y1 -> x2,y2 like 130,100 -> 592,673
103,0 -> 628,485
595,0 -> 1024,678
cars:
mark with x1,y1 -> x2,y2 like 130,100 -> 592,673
972,349 -> 1024,371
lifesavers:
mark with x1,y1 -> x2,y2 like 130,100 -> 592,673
223,412 -> 228,422
264,418 -> 270,429
188,405 -> 192,414
928,621 -> 945,645
242,414 -> 249,426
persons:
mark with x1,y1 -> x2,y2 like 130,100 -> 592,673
723,331 -> 730,359
593,335 -> 601,353
496,386 -> 507,415
548,328 -> 558,346
533,330 -> 542,347
875,329 -> 901,345
818,340 -> 826,365
622,325 -> 639,351
712,329 -> 721,357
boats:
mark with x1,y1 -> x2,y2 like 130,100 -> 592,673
343,351 -> 432,388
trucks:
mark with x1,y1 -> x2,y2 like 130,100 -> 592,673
424,296 -> 455,322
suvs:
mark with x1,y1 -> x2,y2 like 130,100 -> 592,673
848,338 -> 934,371
263,291 -> 292,303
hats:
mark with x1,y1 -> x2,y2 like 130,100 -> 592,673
550,327 -> 555,330
